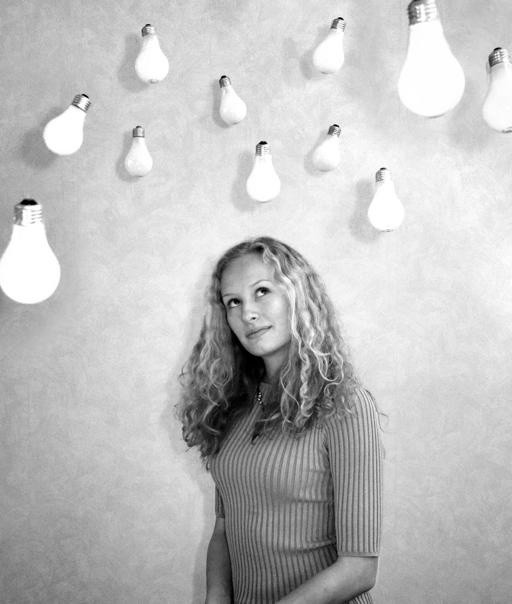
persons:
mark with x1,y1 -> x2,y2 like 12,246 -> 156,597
170,232 -> 389,604
